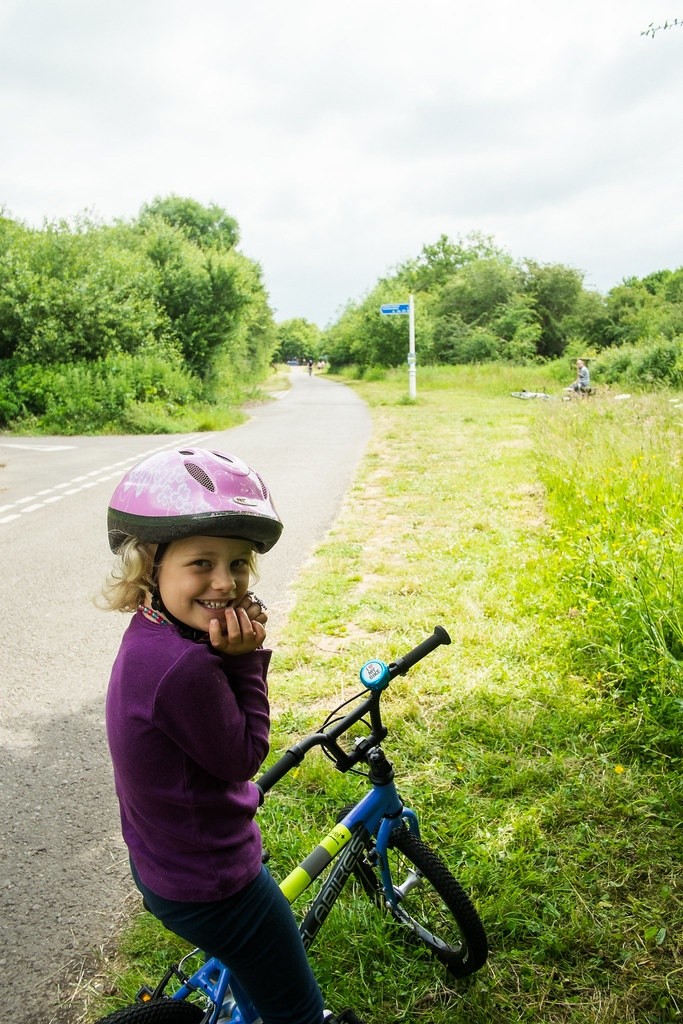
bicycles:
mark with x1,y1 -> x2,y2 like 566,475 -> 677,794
95,626 -> 488,1024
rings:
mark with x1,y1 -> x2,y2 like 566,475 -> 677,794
254,596 -> 268,611
245,590 -> 254,600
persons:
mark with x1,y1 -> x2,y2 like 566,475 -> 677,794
309,359 -> 326,370
570,360 -> 590,392
94,444 -> 360,1024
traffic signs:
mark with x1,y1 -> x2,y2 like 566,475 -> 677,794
378,303 -> 410,315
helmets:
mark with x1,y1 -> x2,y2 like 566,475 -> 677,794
109,447 -> 284,555
